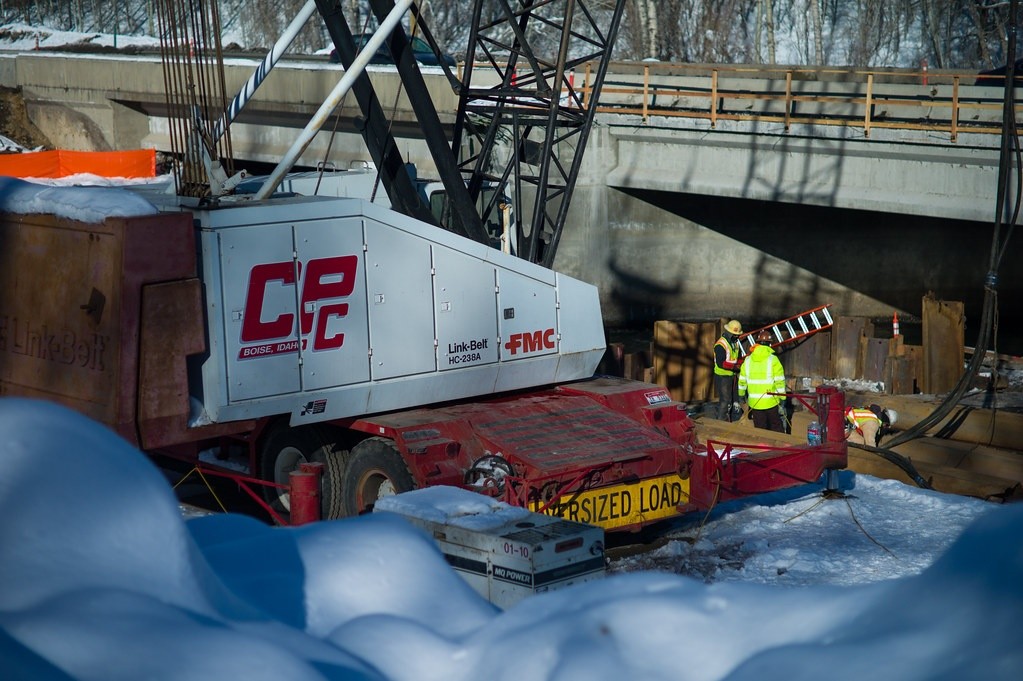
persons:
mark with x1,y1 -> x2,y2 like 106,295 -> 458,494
738,332 -> 787,433
844,404 -> 898,447
713,320 -> 743,422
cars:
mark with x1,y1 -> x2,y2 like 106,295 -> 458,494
329,32 -> 456,66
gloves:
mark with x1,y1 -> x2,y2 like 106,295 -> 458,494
780,400 -> 786,407
739,396 -> 746,403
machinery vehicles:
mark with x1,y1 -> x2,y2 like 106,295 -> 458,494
0,0 -> 856,559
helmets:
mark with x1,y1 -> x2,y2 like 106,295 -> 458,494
756,331 -> 773,342
883,409 -> 898,426
724,320 -> 743,335
